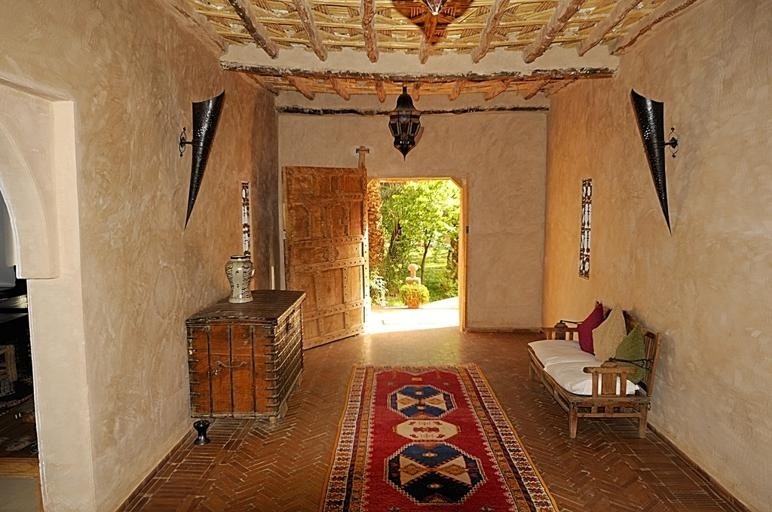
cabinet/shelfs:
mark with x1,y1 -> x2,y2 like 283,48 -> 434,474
185,288 -> 307,425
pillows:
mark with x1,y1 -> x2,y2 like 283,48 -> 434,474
575,303 -> 645,383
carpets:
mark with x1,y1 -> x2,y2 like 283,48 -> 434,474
319,360 -> 559,512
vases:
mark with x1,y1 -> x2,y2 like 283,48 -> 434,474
225,254 -> 254,304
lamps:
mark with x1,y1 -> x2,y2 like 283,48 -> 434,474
177,88 -> 225,227
630,84 -> 679,235
388,80 -> 422,162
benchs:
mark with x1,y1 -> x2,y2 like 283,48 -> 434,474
528,301 -> 663,440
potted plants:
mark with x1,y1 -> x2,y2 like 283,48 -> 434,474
399,281 -> 429,309
